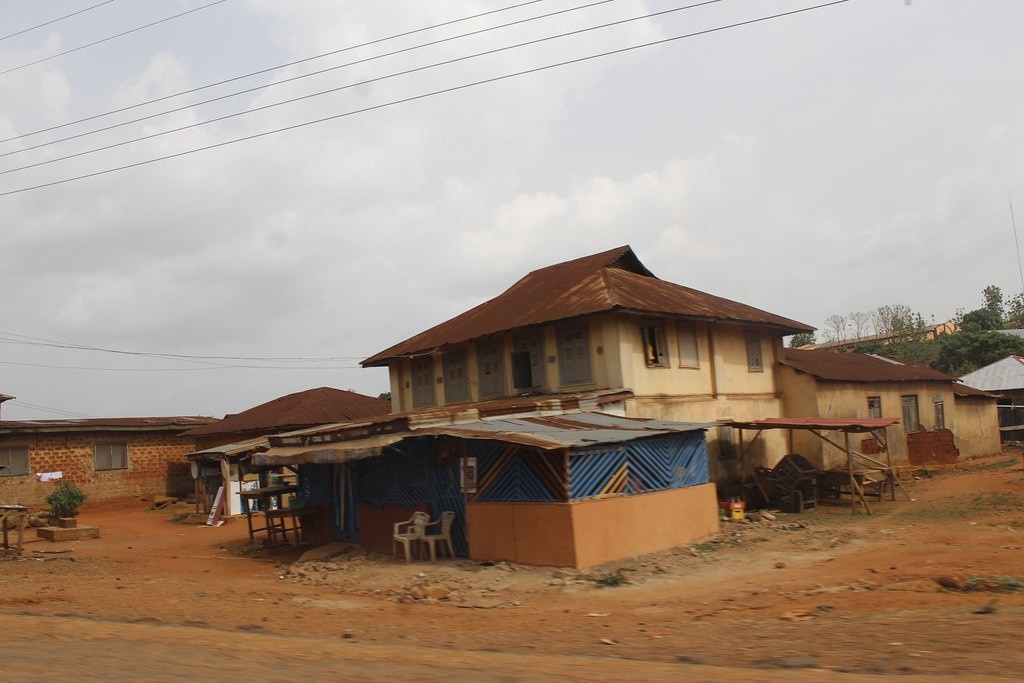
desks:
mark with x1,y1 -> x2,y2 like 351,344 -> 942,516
741,452 -> 885,513
0,505 -> 32,556
235,486 -> 300,541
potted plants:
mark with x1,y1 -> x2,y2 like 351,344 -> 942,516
46,480 -> 87,528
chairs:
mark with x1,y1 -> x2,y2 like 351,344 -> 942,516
392,512 -> 431,564
420,510 -> 456,564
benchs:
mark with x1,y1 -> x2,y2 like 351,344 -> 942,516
268,508 -> 322,551
